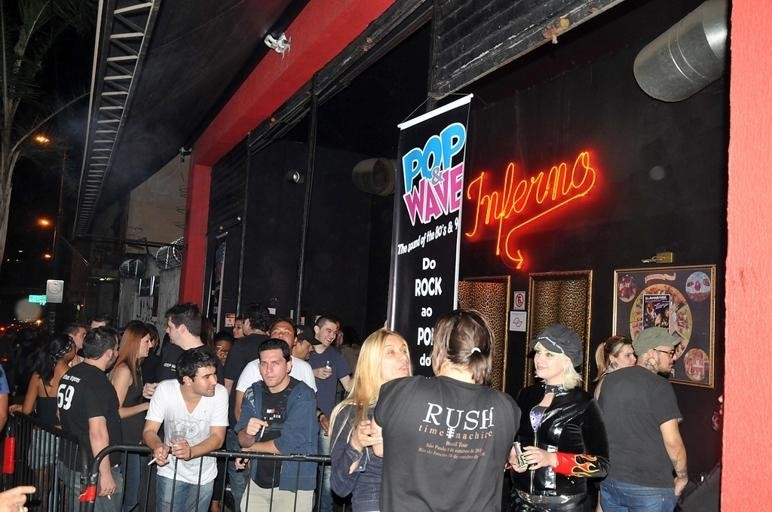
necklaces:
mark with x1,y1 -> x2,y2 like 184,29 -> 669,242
544,384 -> 571,395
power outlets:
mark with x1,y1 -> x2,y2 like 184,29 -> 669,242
641,251 -> 673,263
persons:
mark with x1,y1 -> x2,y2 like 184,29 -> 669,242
504,322 -> 610,511
1,302 -> 355,512
593,335 -> 637,512
594,326 -> 689,512
370,309 -> 523,512
328,327 -> 414,511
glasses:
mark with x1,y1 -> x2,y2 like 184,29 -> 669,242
651,348 -> 676,358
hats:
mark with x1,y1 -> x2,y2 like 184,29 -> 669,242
632,327 -> 682,357
528,324 -> 584,368
295,324 -> 321,346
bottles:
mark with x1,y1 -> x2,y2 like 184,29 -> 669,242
326,361 -> 331,368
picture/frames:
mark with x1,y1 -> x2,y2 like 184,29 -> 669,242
524,269 -> 592,394
459,273 -> 511,393
612,265 -> 717,389
